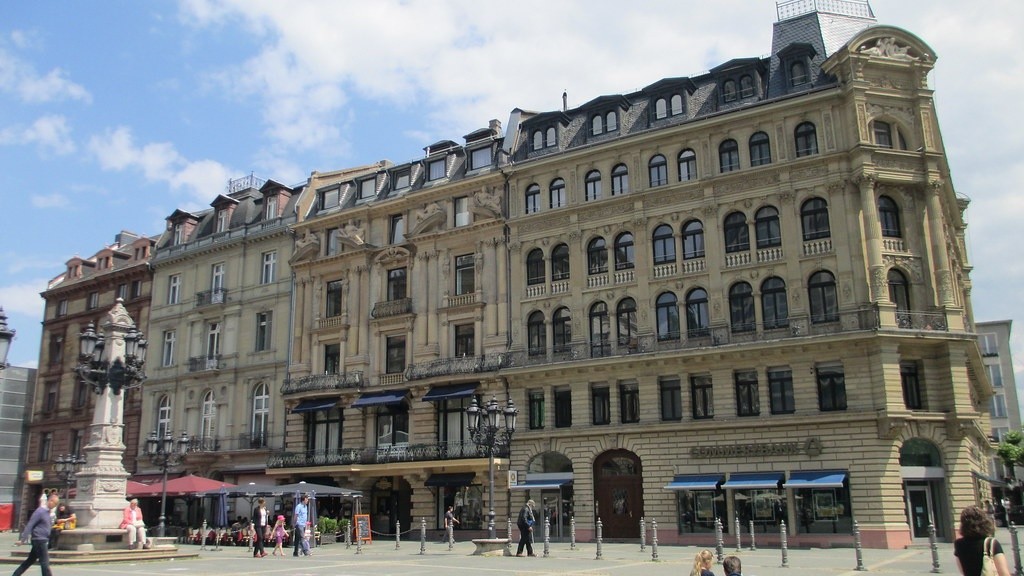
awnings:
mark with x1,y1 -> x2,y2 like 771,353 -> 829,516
508,479 -> 572,489
720,473 -> 782,489
782,471 -> 846,488
973,471 -> 996,486
290,398 -> 337,418
424,472 -> 475,496
421,383 -> 477,408
663,475 -> 721,490
351,390 -> 406,415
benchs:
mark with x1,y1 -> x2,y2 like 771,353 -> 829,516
56,525 -> 148,551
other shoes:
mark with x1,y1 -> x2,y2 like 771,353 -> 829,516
528,553 -> 537,557
293,553 -> 299,557
49,546 -> 58,550
261,552 -> 268,557
281,554 -> 285,557
254,553 -> 262,558
516,554 -> 525,557
129,545 -> 133,549
272,552 -> 277,556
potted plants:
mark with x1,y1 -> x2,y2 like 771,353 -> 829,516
316,516 -> 353,544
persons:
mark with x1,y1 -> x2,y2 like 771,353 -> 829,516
342,219 -> 358,234
231,494 -> 313,557
119,499 -> 147,550
681,503 -> 813,534
516,500 -> 536,557
10,495 -> 77,575
444,505 -> 460,548
954,500 -> 1010,576
476,186 -> 501,214
859,37 -> 919,60
690,549 -> 741,576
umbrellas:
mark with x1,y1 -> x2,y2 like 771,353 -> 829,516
65,472 -> 363,548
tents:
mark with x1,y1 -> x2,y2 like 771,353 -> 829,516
711,492 -> 803,502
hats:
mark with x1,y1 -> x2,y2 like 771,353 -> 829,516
278,516 -> 285,521
306,521 -> 311,525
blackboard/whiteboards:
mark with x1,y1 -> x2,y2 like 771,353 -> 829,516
352,514 -> 372,540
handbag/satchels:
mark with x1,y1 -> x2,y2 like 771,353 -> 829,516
980,537 -> 999,576
134,520 -> 145,528
